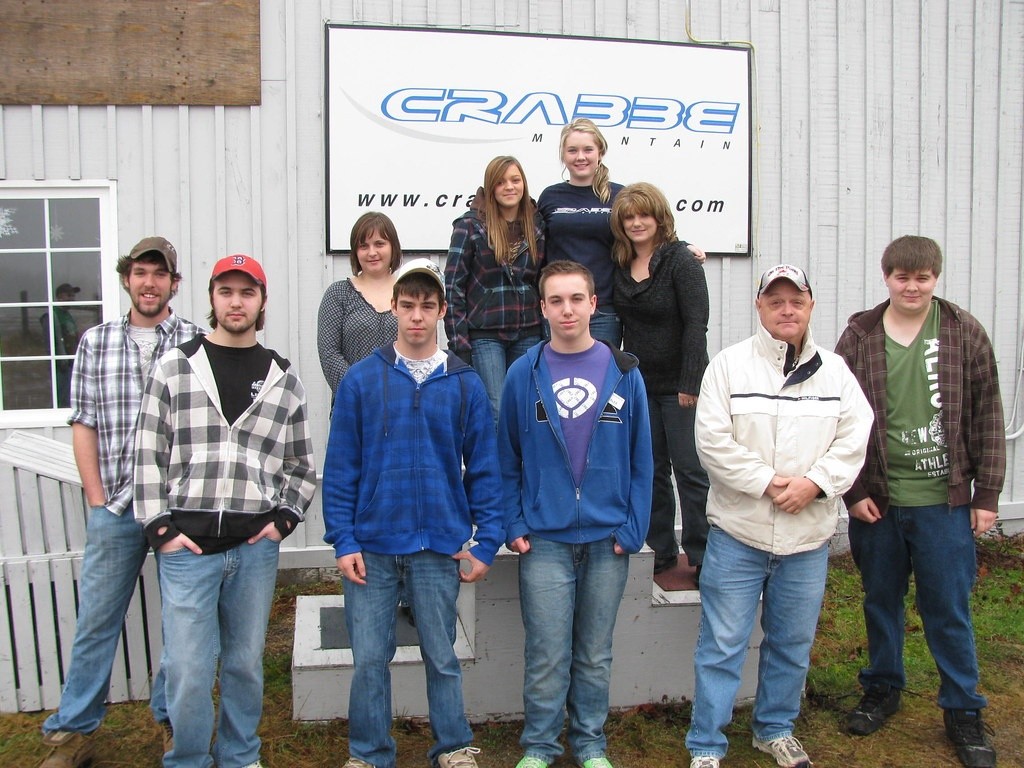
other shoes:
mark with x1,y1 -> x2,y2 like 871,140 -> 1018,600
39,729 -> 97,768
695,565 -> 703,583
652,553 -> 677,575
159,720 -> 176,754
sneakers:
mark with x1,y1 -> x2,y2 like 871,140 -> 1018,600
689,753 -> 720,768
943,705 -> 998,768
341,757 -> 376,768
841,685 -> 900,736
437,746 -> 481,768
751,729 -> 813,768
514,756 -> 547,768
575,755 -> 613,768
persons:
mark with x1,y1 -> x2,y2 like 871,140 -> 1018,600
322,257 -> 507,768
609,181 -> 710,585
443,156 -> 546,429
537,116 -> 706,350
499,261 -> 653,768
834,236 -> 1006,768
685,264 -> 875,768
40,283 -> 81,408
39,236 -> 210,768
317,212 -> 403,421
132,255 -> 317,768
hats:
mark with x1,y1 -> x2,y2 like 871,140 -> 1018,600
392,258 -> 446,298
210,254 -> 266,296
757,264 -> 813,300
128,236 -> 177,273
